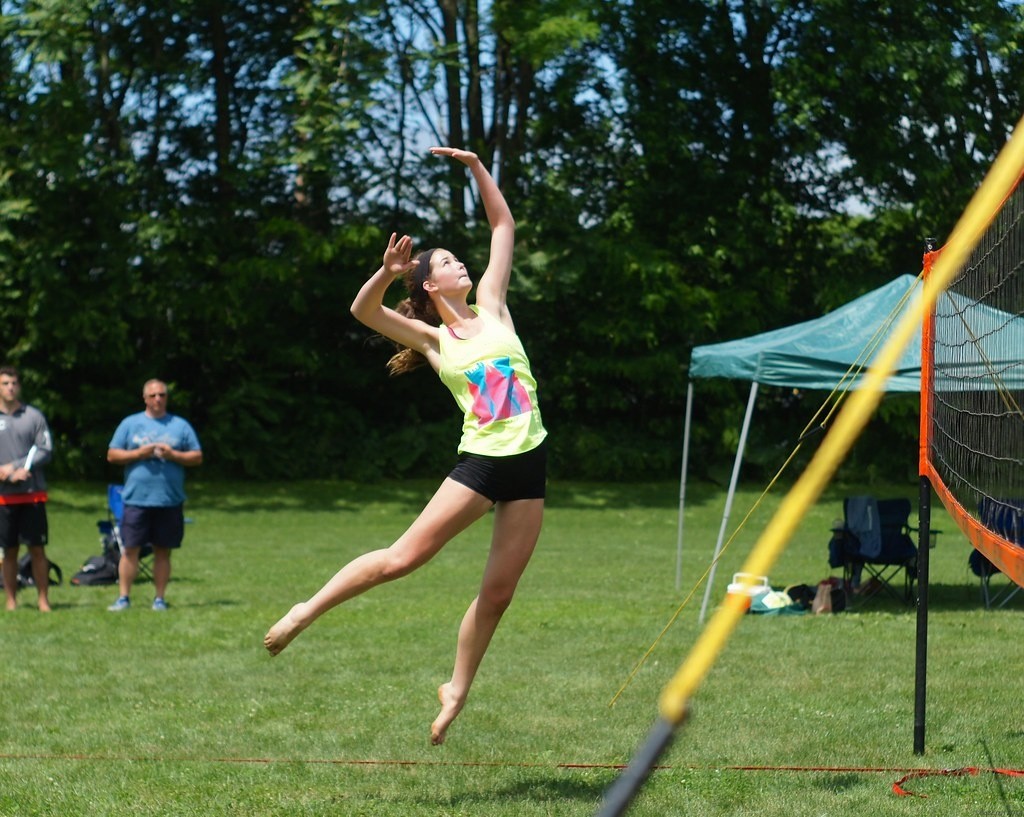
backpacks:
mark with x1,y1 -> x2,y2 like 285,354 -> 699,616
0,549 -> 63,590
71,554 -> 118,586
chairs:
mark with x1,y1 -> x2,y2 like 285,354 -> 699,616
969,497 -> 1024,609
828,496 -> 944,613
107,483 -> 196,584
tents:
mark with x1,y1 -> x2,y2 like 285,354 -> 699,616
672,273 -> 1024,625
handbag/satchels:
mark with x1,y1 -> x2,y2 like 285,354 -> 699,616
728,573 -> 847,615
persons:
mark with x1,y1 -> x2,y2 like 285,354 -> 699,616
0,368 -> 55,613
104,378 -> 203,611
262,145 -> 552,747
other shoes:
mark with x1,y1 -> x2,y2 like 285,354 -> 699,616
151,598 -> 167,612
106,595 -> 130,612
